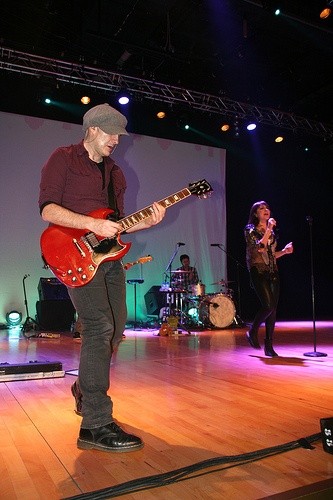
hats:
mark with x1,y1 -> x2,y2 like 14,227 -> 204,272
83,103 -> 130,136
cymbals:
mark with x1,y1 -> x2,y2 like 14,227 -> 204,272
167,270 -> 190,273
210,281 -> 235,285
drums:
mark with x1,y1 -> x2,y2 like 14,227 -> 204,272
186,284 -> 205,302
199,294 -> 236,328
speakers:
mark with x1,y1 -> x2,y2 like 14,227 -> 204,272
144,285 -> 169,315
36,301 -> 75,331
37,277 -> 71,300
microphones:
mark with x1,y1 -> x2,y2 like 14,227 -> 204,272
213,303 -> 219,308
210,244 -> 223,246
177,242 -> 185,246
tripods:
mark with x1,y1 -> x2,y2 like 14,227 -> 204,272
21,276 -> 36,333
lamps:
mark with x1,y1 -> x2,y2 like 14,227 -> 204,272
6,311 -> 22,326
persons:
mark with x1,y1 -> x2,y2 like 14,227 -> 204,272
39,104 -> 166,455
245,201 -> 293,357
173,254 -> 201,295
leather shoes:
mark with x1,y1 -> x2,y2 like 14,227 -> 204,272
70,381 -> 83,415
78,422 -> 145,453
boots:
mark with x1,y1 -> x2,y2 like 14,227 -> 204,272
246,325 -> 261,348
264,335 -> 278,356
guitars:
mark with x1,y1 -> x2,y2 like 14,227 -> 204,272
123,255 -> 152,270
41,179 -> 215,288
